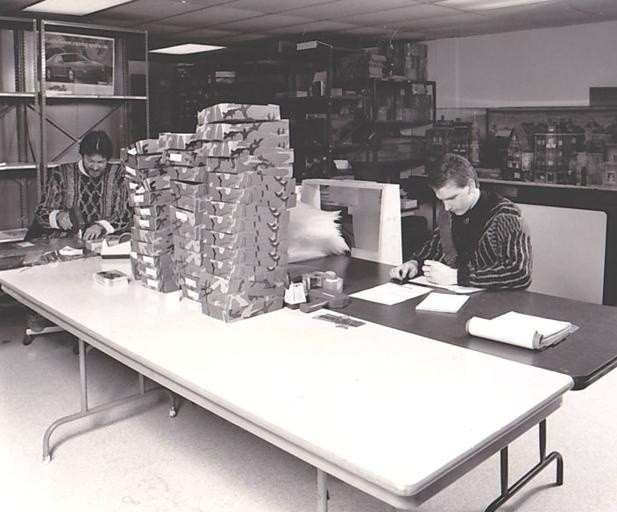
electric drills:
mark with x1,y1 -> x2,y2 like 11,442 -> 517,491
69,205 -> 85,240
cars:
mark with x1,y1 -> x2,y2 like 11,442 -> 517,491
45,52 -> 107,83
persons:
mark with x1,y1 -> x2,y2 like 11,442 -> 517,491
31,130 -> 131,241
385,152 -> 534,293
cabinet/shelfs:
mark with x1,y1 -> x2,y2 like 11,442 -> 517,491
0,15 -> 43,309
13,14 -> 153,246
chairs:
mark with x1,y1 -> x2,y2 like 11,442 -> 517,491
16,189 -> 97,358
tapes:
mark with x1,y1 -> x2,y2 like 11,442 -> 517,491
284,269 -> 301,289
325,270 -> 336,280
118,232 -> 131,244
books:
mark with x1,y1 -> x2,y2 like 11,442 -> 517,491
465,309 -> 581,352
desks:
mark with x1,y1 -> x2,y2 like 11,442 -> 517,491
0,252 -> 576,510
286,253 -> 616,393
